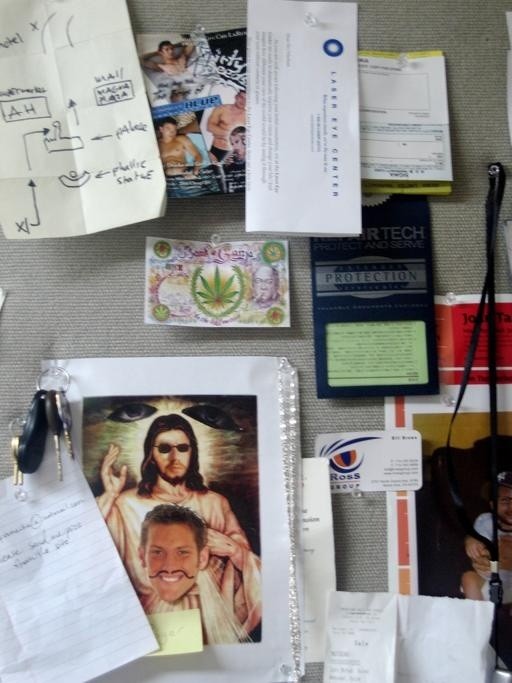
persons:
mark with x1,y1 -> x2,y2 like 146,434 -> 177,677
140,504 -> 209,604
138,38 -> 197,103
220,123 -> 246,190
156,115 -> 203,195
248,263 -> 283,309
91,413 -> 263,648
462,471 -> 512,658
206,89 -> 246,162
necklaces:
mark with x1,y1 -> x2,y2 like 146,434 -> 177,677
274,355 -> 308,682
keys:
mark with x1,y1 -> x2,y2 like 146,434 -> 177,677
8,365 -> 78,489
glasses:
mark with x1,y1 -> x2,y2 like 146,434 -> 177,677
152,443 -> 191,453
